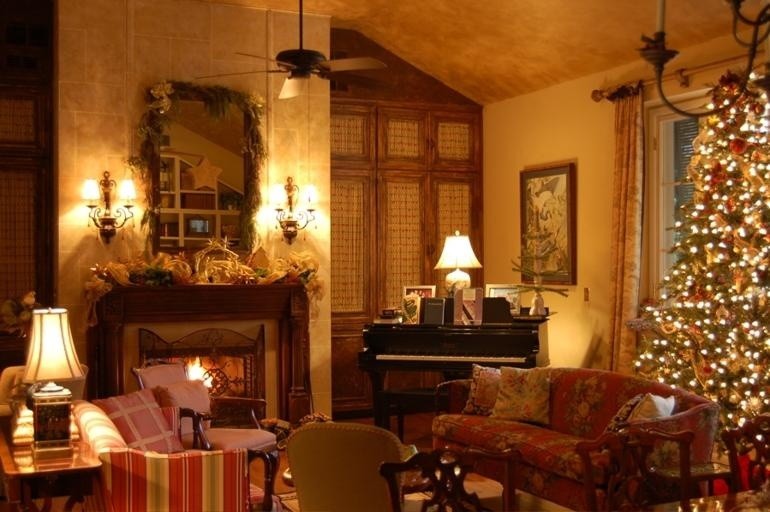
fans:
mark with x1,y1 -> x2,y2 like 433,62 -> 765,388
188,2 -> 388,102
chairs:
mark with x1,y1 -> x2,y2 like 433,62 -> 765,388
721,410 -> 770,488
276,421 -> 409,512
133,361 -> 289,512
76,386 -> 255,512
376,444 -> 518,512
566,425 -> 692,512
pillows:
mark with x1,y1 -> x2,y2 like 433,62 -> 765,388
460,361 -> 501,416
600,392 -> 674,441
491,365 -> 553,427
156,380 -> 212,433
93,389 -> 183,456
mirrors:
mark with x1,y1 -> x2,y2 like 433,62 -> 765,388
148,81 -> 253,250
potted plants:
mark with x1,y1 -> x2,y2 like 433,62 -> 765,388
511,227 -> 567,317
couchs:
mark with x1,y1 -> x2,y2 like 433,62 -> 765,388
427,362 -> 718,512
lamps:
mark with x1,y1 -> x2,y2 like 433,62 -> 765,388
22,307 -> 86,456
633,0 -> 770,117
434,228 -> 483,298
79,169 -> 138,246
265,174 -> 316,244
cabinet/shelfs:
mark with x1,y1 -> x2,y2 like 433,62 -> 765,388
2,79 -> 54,350
331,323 -> 372,419
331,103 -> 373,321
374,103 -> 481,321
159,149 -> 245,246
385,371 -> 445,418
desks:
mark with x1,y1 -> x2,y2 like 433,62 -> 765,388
648,462 -> 731,501
653,489 -> 769,511
0,401 -> 102,512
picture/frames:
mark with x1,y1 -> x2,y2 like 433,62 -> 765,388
521,161 -> 576,285
403,284 -> 436,324
488,283 -> 521,315
